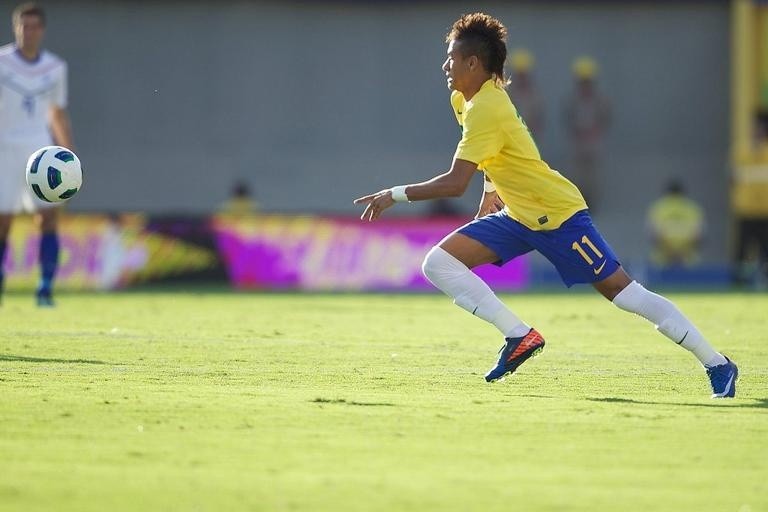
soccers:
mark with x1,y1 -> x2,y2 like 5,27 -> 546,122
25,146 -> 82,203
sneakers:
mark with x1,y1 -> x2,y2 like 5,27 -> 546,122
485,327 -> 545,383
704,356 -> 738,399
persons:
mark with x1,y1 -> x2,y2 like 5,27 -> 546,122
726,106 -> 767,280
1,0 -> 69,306
504,50 -> 546,149
555,54 -> 614,215
644,172 -> 707,279
220,180 -> 256,212
353,13 -> 738,398
431,197 -> 463,216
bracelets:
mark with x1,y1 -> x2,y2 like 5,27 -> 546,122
391,184 -> 411,203
